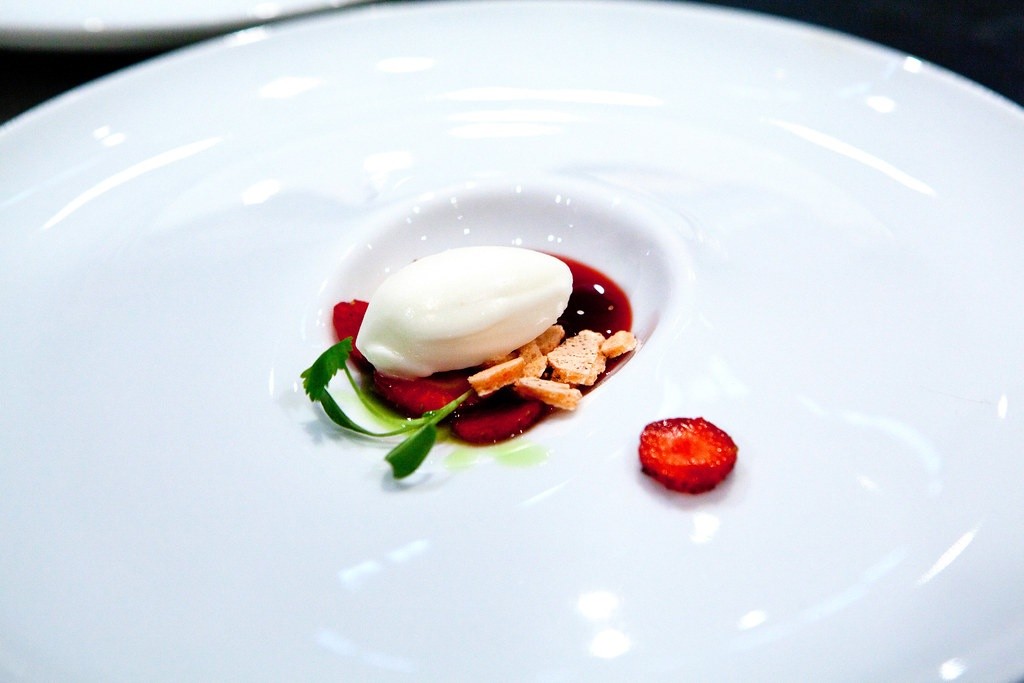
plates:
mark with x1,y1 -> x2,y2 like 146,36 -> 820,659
0,0 -> 1024,683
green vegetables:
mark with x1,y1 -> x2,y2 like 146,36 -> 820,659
301,335 -> 474,479
